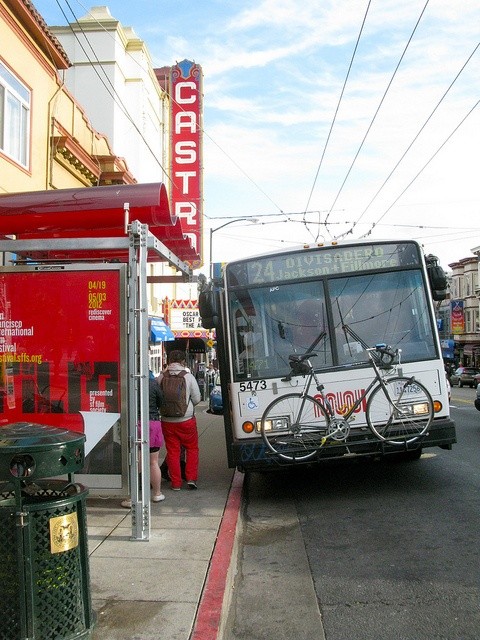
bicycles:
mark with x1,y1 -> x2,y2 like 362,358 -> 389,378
260,342 -> 435,465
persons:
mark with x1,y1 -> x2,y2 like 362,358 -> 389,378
121,370 -> 165,507
158,351 -> 201,490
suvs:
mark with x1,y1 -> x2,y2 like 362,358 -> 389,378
449,367 -> 480,389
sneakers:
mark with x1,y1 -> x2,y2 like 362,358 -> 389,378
187,480 -> 197,488
121,500 -> 131,508
173,487 -> 180,490
153,494 -> 165,502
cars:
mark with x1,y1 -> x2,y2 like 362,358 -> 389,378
209,370 -> 224,414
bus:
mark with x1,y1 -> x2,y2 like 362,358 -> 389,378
198,238 -> 458,479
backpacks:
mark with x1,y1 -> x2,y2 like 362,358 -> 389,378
159,370 -> 191,417
